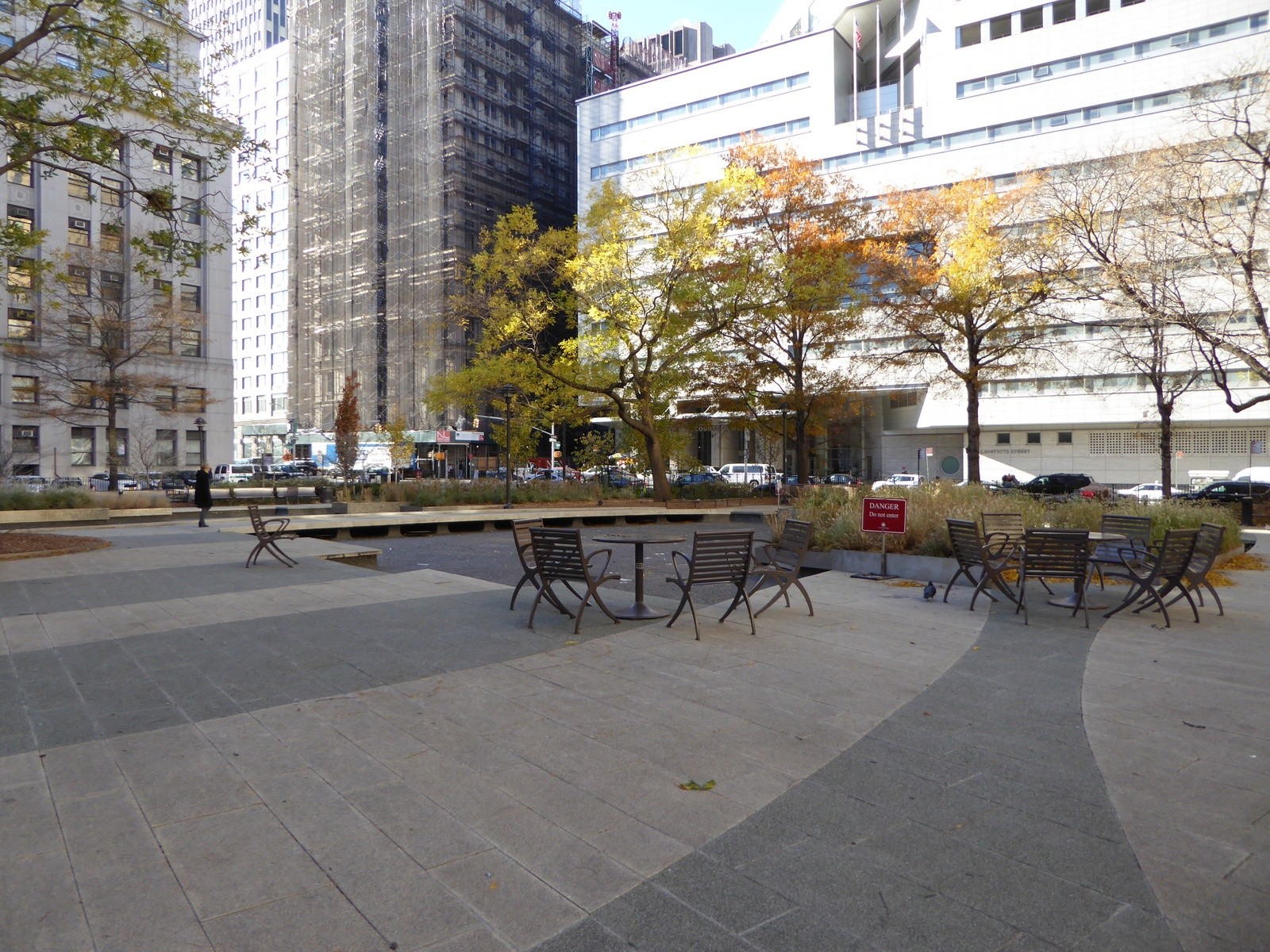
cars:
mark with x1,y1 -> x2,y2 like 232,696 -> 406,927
6,470 -> 210,493
1116,481 -> 1186,502
484,466 -> 726,489
957,479 -> 999,490
755,473 -> 863,496
253,464 -> 308,480
317,464 -> 354,479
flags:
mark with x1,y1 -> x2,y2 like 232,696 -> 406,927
879,12 -> 884,33
855,20 -> 862,50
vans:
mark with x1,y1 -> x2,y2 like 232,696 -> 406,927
716,462 -> 777,490
871,473 -> 926,491
214,463 -> 256,484
1234,465 -> 1270,483
235,458 -> 268,472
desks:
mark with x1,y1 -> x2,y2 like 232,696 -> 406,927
1030,530 -> 1125,610
592,533 -> 686,620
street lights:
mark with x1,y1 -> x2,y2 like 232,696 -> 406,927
917,445 -> 923,483
195,417 -> 207,471
499,384 -> 518,509
780,401 -> 791,486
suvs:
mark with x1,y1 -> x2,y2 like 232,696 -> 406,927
358,464 -> 390,485
1172,481 -> 1270,506
283,457 -> 318,476
1017,474 -> 1095,503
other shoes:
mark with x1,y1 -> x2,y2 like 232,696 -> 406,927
198,521 -> 210,527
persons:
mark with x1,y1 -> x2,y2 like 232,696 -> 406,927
194,463 -> 214,527
825,477 -> 831,484
458,461 -> 463,479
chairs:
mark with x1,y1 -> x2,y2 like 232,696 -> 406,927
980,512 -> 1055,597
666,530 -> 756,641
1132,520 -> 1226,616
1083,514 -> 1153,601
735,518 -> 815,617
245,505 -> 299,568
943,517 -> 1025,611
527,526 -> 621,635
1102,527 -> 1200,627
508,517 -> 593,614
1016,528 -> 1090,629
